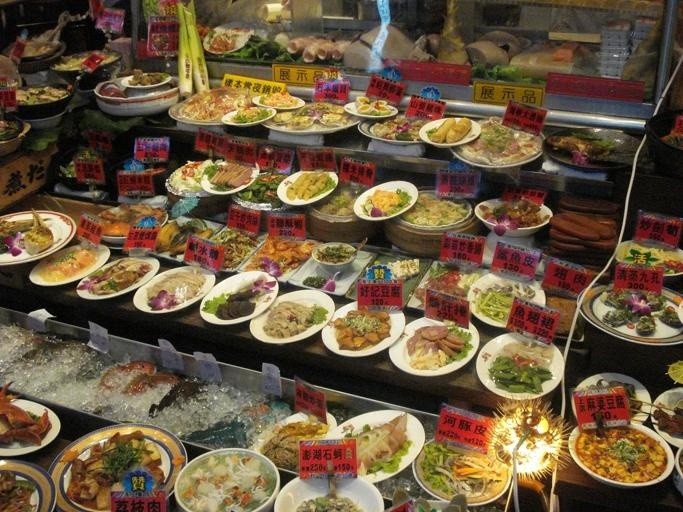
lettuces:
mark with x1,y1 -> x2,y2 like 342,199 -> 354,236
440,327 -> 472,365
421,441 -> 455,495
345,425 -> 412,474
204,294 -> 232,315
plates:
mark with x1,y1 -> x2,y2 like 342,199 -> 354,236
76,252 -> 161,300
407,260 -> 490,312
133,265 -> 216,314
397,189 -> 472,227
199,270 -> 279,326
121,73 -> 172,89
450,121 -> 546,169
97,206 -> 169,245
0,210 -> 77,266
28,241 -> 111,288
249,288 -> 336,345
238,238 -> 325,284
476,332 -> 565,401
150,216 -> 224,262
357,111 -> 424,145
202,21 -> 254,55
571,371 -> 652,425
353,180 -> 419,222
576,280 -> 683,347
276,170 -> 339,207
0,459 -> 57,512
466,273 -> 546,328
0,399 -> 61,457
615,239 -> 683,277
252,93 -> 306,110
412,438 -> 512,507
542,127 -> 648,172
321,300 -> 406,358
49,423 -> 188,512
650,387 -> 683,449
222,107 -> 276,127
273,474 -> 385,512
202,160 -> 259,195
260,121 -> 360,135
255,410 -> 338,472
324,409 -> 426,484
418,116 -> 482,149
388,316 -> 480,378
288,250 -> 378,297
168,99 -> 222,127
344,101 -> 399,118
210,227 -> 269,272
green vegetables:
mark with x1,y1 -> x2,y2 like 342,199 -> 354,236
98,439 -> 144,481
477,290 -> 517,324
302,276 -> 326,288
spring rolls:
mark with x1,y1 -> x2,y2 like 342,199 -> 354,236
446,118 -> 472,143
430,117 -> 454,143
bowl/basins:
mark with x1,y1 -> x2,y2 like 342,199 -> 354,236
24,112 -> 65,129
174,447 -> 280,512
51,49 -> 123,90
313,242 -> 358,274
4,41 -> 66,73
14,83 -> 75,118
672,448 -> 683,495
0,119 -> 32,157
94,78 -> 180,117
647,110 -> 683,176
474,197 -> 554,238
567,421 -> 674,489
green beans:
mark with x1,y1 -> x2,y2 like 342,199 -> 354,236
490,357 -> 551,393
